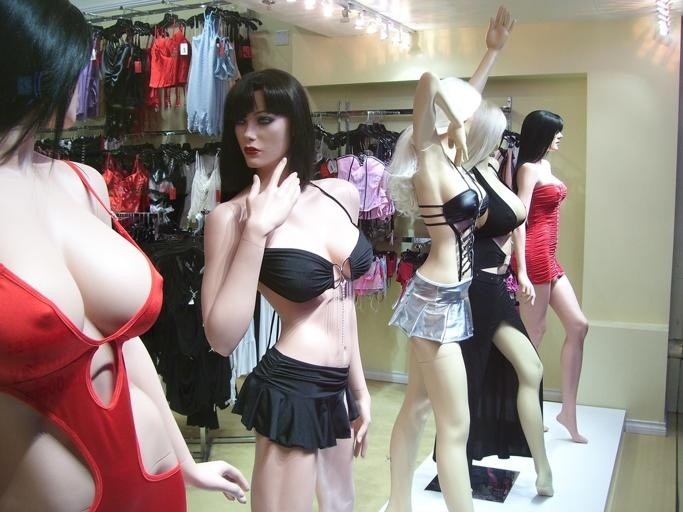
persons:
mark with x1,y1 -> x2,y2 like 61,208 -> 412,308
380,4 -> 515,511
431,102 -> 554,500
505,112 -> 591,446
199,67 -> 374,512
0,2 -> 250,511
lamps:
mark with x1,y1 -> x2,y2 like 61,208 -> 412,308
655,0 -> 673,38
